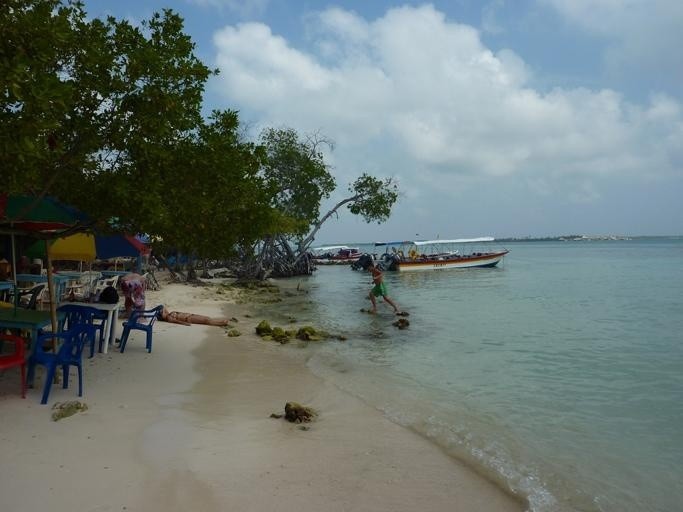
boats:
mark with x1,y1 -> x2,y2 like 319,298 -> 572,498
358,236 -> 512,273
306,246 -> 363,265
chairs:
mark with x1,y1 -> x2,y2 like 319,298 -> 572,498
0,269 -> 164,405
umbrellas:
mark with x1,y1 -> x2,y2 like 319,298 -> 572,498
0,194 -> 163,384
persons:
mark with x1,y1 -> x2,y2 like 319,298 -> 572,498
366,264 -> 400,313
157,308 -> 228,326
119,258 -> 147,323
